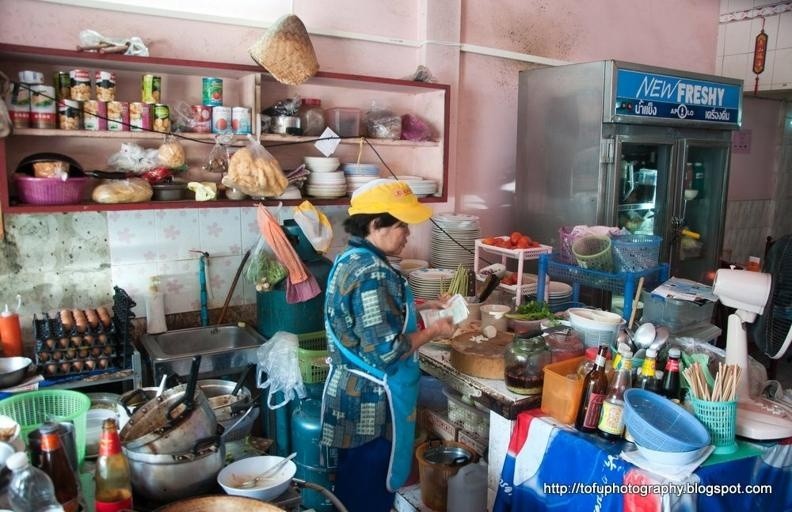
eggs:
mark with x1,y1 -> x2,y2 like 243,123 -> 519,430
40,307 -> 112,374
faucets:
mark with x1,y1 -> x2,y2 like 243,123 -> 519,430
188,250 -> 214,300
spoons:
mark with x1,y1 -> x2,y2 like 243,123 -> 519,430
235,451 -> 298,490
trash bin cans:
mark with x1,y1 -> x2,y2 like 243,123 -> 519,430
416,441 -> 479,510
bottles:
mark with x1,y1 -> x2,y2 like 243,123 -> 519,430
1,294 -> 23,356
621,151 -> 659,197
503,330 -> 552,396
92,417 -> 134,511
574,344 -> 683,447
38,424 -> 79,511
685,157 -> 707,191
4,448 -> 66,511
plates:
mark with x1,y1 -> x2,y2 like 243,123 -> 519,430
531,279 -> 574,305
429,211 -> 482,271
307,172 -> 437,200
407,267 -> 457,302
390,256 -> 428,278
83,390 -> 129,459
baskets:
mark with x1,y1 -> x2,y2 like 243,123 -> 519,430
568,308 -> 627,346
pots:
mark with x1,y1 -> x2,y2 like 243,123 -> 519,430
118,354 -> 264,501
14,152 -> 128,180
421,435 -> 474,466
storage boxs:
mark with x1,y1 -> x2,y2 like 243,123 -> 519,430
326,106 -> 360,138
641,288 -> 716,335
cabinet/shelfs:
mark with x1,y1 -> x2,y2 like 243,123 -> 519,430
473,235 -> 554,308
538,250 -> 669,322
0,41 -> 450,213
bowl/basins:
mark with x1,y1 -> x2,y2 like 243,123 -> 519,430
683,188 -> 699,199
303,156 -> 342,171
265,183 -> 303,200
216,455 -> 297,503
151,181 -> 189,201
568,307 -> 627,331
539,312 -> 571,338
590,310 -> 621,322
225,187 -> 246,200
0,356 -> 32,390
343,163 -> 381,176
510,307 -> 545,329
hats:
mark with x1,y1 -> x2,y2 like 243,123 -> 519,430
349,180 -> 433,224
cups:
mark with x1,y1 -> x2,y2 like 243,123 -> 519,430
479,304 -> 511,331
29,423 -> 77,477
466,304 -> 483,320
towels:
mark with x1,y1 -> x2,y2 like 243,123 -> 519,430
253,203 -> 323,306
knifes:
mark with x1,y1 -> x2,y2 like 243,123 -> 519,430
476,274 -> 501,303
465,269 -> 478,302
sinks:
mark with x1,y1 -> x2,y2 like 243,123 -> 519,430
139,321 -> 268,387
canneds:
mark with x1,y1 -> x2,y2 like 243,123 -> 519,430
4,68 -> 172,133
192,76 -> 251,134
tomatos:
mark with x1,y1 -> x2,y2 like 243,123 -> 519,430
483,231 -> 540,249
501,272 -> 519,285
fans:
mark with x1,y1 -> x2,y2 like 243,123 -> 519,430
711,233 -> 792,441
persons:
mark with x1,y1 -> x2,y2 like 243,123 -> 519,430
318,179 -> 457,512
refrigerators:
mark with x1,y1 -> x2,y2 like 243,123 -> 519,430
517,55 -> 745,271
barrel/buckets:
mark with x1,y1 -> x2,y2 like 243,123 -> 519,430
566,307 -> 627,354
